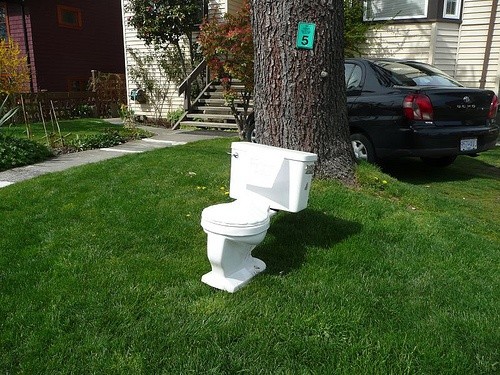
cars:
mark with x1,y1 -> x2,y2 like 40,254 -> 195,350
246,58 -> 500,168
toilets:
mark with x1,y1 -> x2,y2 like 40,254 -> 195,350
199,139 -> 319,295
